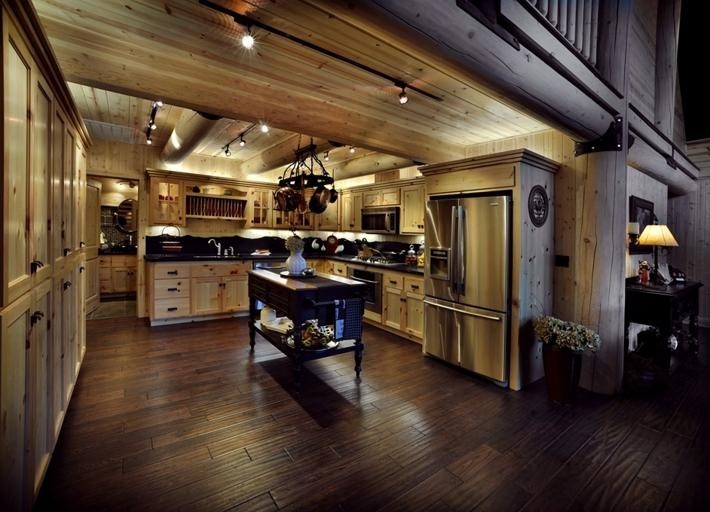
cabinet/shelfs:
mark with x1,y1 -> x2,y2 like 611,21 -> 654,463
381,269 -> 425,346
144,262 -> 190,326
98,255 -> 138,297
190,258 -> 254,323
0,0 -> 93,512
148,176 -> 427,235
306,257 -> 349,278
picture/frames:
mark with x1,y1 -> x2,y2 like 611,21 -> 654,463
528,184 -> 549,227
629,194 -> 655,254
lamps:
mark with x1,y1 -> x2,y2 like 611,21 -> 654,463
635,224 -> 679,290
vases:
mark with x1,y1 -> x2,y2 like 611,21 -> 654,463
286,247 -> 306,274
542,340 -> 583,405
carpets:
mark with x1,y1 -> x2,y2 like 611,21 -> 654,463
87,300 -> 136,319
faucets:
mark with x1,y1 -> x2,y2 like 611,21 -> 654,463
207,238 -> 221,255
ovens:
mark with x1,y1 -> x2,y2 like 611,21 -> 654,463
347,267 -> 382,314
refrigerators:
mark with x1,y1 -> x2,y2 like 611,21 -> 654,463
423,195 -> 511,383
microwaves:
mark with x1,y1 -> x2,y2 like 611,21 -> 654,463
361,207 -> 396,234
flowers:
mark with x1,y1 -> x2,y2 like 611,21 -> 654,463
533,315 -> 602,352
285,236 -> 304,250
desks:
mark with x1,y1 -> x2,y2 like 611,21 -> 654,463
625,273 -> 704,393
245,266 -> 368,395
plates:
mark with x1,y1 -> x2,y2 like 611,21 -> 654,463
280,270 -> 317,278
286,335 -> 338,351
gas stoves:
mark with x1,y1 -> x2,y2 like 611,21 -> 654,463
352,255 -> 408,267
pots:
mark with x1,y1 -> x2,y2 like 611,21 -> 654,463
273,168 -> 338,214
369,248 -> 405,259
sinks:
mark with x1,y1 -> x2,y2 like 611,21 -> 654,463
195,255 -> 237,258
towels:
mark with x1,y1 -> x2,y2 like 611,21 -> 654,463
626,322 -> 654,353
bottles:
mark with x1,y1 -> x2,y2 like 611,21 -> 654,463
641,261 -> 650,286
